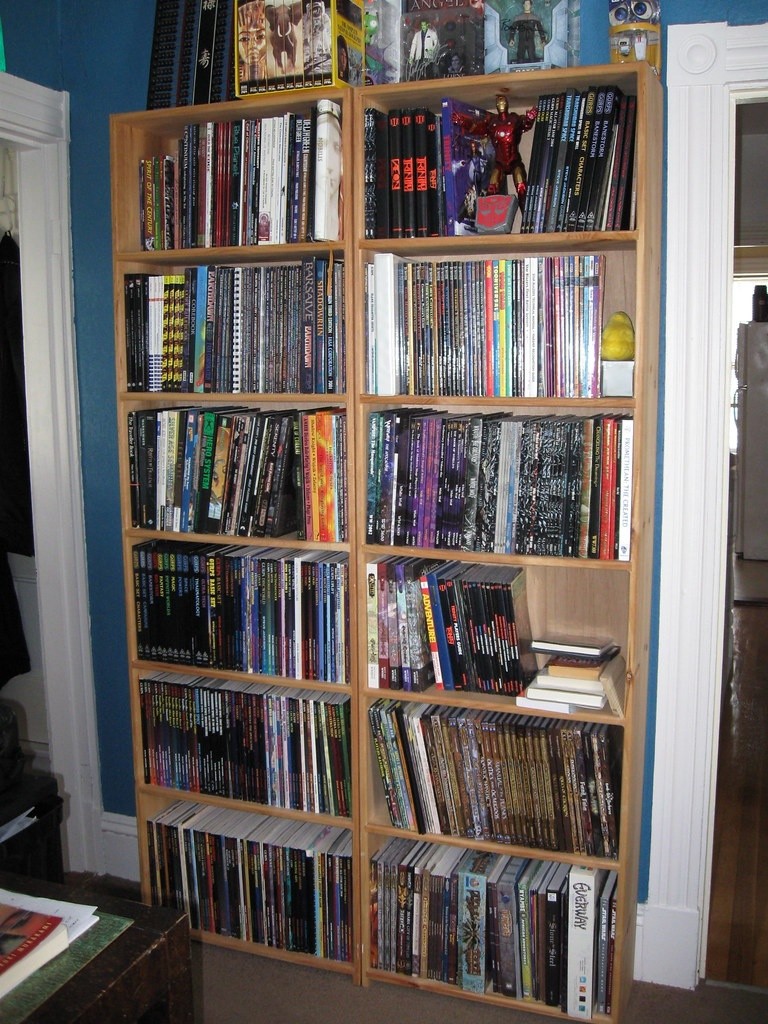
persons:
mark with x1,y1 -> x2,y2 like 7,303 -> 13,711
508,0 -> 546,64
409,20 -> 439,80
451,94 -> 540,230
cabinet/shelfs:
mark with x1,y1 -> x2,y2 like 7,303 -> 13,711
110,60 -> 668,1024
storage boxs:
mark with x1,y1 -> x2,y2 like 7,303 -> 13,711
234,0 -> 365,100
458,848 -> 501,994
566,865 -> 606,1019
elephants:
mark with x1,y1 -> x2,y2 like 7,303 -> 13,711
264,0 -> 302,74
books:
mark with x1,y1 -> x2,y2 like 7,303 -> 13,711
123,89 -> 633,1024
145,0 -> 366,110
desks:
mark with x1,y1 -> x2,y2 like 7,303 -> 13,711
0,774 -> 65,885
0,870 -> 196,1024
